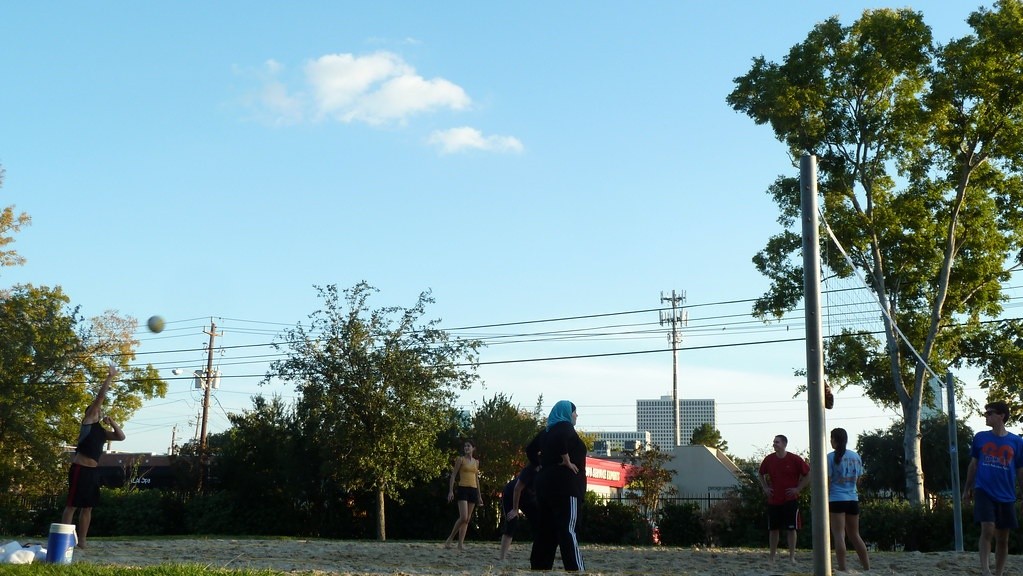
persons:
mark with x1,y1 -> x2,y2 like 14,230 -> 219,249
829,428 -> 870,571
526,400 -> 589,572
961,401 -> 1023,576
62,360 -> 125,548
759,435 -> 809,561
500,461 -> 542,563
445,441 -> 481,551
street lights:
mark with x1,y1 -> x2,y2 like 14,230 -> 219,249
172,370 -> 212,449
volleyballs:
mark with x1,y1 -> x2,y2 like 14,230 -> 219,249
148,316 -> 165,333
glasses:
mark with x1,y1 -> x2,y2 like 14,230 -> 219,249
984,411 -> 998,417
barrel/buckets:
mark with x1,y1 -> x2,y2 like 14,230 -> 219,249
46,523 -> 79,564
